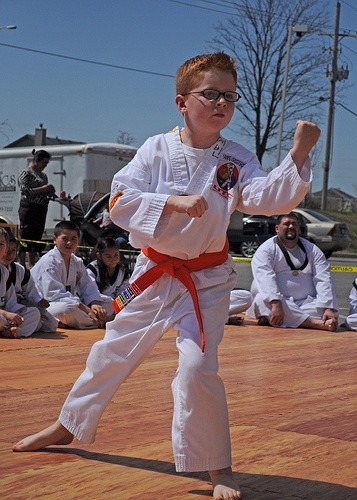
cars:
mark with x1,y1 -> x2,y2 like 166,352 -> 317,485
269,207 -> 350,259
223,218 -> 285,257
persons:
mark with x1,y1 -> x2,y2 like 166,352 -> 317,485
224,289 -> 252,325
245,214 -> 338,330
85,238 -> 132,300
10,51 -> 320,500
29,221 -> 115,330
17,148 -> 55,268
339,276 -> 356,332
0,237 -> 58,333
0,228 -> 40,338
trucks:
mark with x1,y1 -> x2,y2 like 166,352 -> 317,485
0,141 -> 142,240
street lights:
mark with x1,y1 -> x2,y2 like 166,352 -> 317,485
274,22 -> 312,168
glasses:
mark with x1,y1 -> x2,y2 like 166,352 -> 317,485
182,90 -> 241,103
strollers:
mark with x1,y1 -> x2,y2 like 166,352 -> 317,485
48,189 -> 130,249
